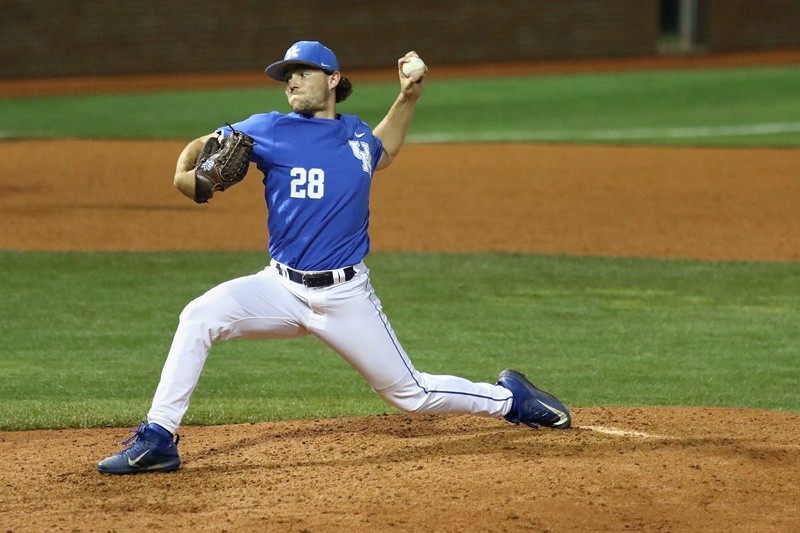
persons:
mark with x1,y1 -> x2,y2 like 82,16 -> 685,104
95,41 -> 572,474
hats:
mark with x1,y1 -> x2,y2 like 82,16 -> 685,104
265,41 -> 338,81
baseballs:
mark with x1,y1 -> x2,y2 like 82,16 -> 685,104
402,58 -> 425,81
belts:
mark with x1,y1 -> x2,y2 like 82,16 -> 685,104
275,263 -> 356,287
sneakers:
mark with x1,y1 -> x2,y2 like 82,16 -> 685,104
97,424 -> 181,474
496,368 -> 571,429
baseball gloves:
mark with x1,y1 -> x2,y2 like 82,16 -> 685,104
194,129 -> 255,204
